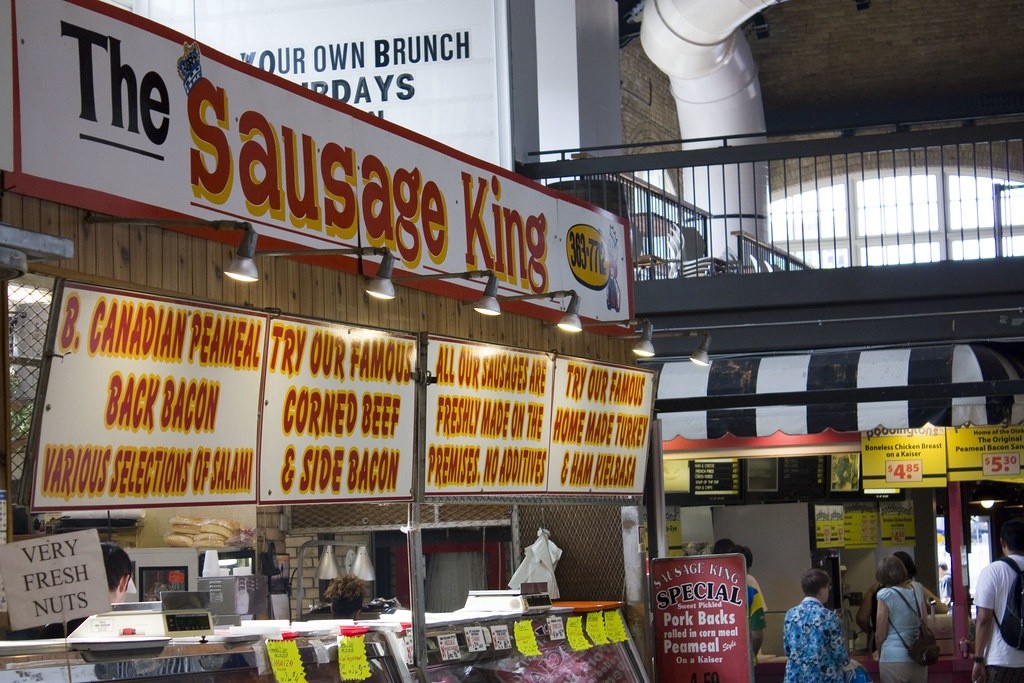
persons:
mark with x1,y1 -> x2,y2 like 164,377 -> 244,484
857,551 -> 943,683
713,539 -> 768,666
782,569 -> 849,683
39,541 -> 132,639
971,521 -> 1024,683
938,563 -> 952,608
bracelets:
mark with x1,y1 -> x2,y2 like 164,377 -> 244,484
974,656 -> 983,663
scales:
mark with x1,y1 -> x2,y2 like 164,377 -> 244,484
66,590 -> 216,644
464,582 -> 552,614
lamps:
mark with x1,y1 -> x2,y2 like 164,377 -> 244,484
258,246 -> 395,301
968,480 -> 1007,509
620,328 -> 712,367
396,269 -> 501,316
87,218 -> 259,283
582,317 -> 655,357
499,290 -> 582,333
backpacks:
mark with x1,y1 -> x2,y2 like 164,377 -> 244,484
887,584 -> 941,666
992,556 -> 1024,651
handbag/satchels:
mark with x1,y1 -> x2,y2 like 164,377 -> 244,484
845,664 -> 873,683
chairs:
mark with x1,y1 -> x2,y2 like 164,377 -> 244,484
667,221 -> 728,279
